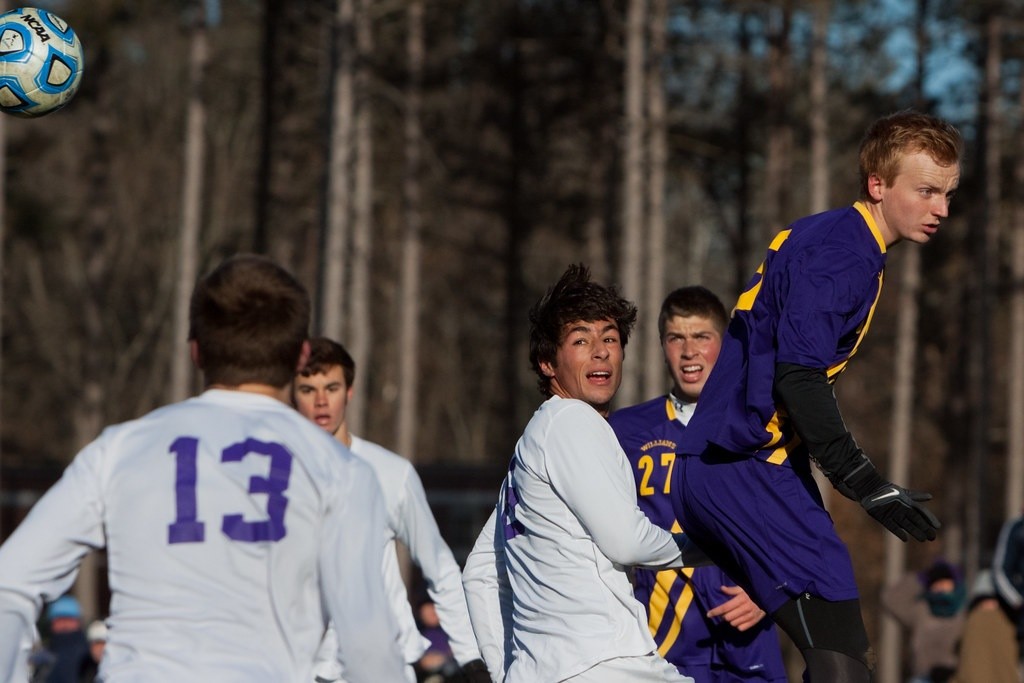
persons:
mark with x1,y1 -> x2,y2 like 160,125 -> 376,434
669,110 -> 964,683
409,262 -> 790,683
30,597 -> 108,682
883,513 -> 1024,683
0,252 -> 409,683
291,336 -> 493,683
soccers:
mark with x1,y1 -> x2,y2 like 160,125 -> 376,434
0,8 -> 87,117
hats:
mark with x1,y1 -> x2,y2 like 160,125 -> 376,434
48,594 -> 80,623
87,620 -> 110,643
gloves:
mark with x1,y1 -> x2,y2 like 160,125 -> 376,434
463,659 -> 492,683
846,461 -> 942,543
